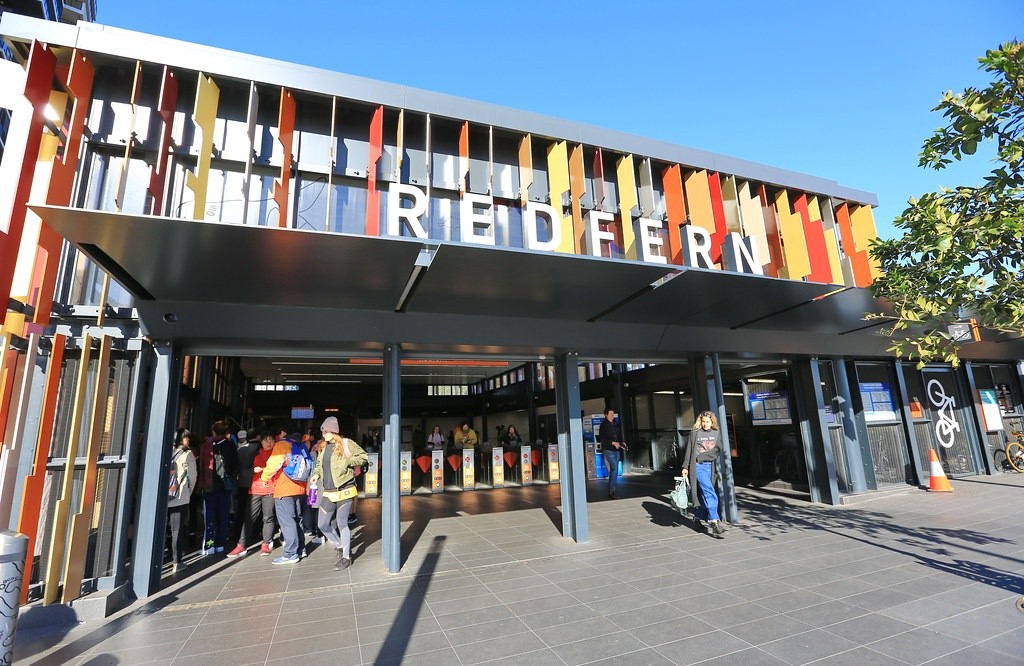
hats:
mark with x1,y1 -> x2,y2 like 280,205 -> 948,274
237,430 -> 247,438
320,416 -> 339,433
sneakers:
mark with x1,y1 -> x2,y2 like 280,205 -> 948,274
333,557 -> 353,571
336,547 -> 343,559
298,548 -> 307,558
311,536 -> 325,544
271,552 -> 299,565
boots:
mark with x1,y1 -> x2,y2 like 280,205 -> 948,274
608,489 -> 621,500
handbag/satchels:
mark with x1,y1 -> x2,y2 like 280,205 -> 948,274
669,473 -> 691,516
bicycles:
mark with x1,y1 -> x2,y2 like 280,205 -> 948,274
1005,421 -> 1024,473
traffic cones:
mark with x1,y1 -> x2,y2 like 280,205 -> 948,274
926,449 -> 955,492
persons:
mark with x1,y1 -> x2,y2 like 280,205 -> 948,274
165,421 -> 382,558
167,427 -> 198,573
260,423 -> 307,565
599,407 -> 628,499
125,430 -> 145,568
681,411 -> 728,533
413,421 -> 521,482
310,416 -> 368,571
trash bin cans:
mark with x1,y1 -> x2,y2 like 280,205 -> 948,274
0,530 -> 29,666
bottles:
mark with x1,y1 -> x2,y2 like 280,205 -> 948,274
307,481 -> 318,505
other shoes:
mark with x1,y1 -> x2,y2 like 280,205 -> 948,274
690,517 -> 728,535
226,543 -> 247,557
260,543 -> 270,556
173,562 -> 187,572
304,529 -> 318,536
198,547 -> 215,555
215,547 -> 224,552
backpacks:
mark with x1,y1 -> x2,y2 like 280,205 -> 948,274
280,438 -> 314,482
168,449 -> 187,501
